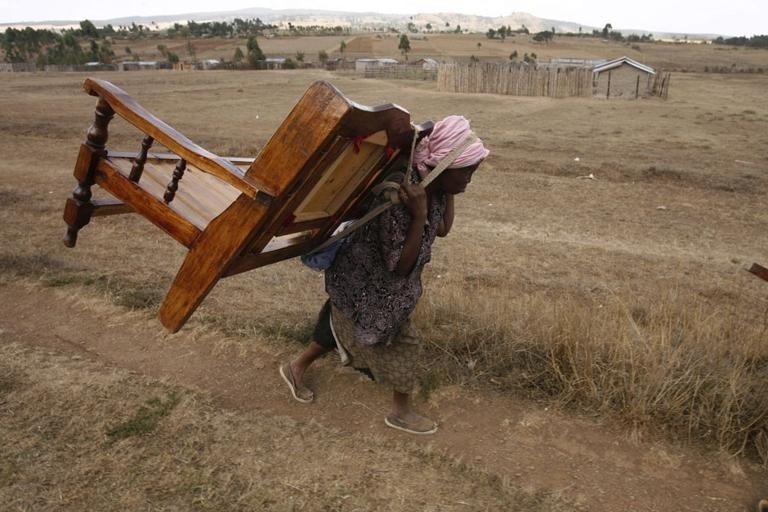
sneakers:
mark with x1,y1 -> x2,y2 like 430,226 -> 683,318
279,362 -> 313,403
385,412 -> 438,434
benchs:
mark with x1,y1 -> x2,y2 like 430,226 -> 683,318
63,77 -> 435,334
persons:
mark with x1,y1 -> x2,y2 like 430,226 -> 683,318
278,115 -> 490,435
